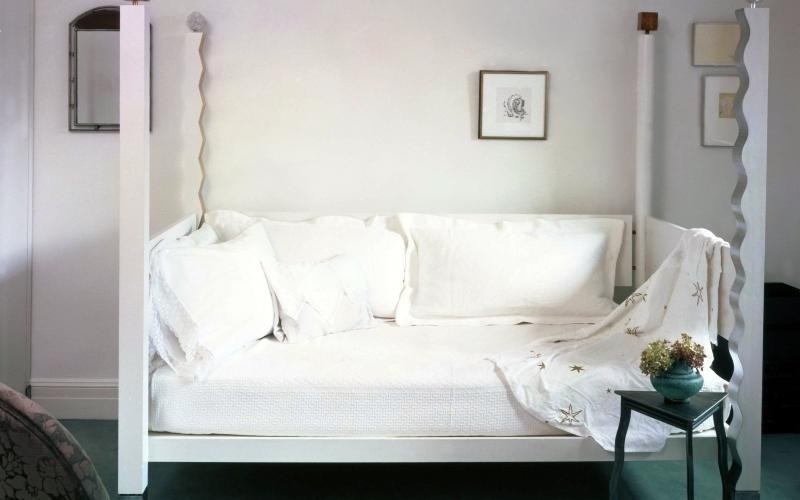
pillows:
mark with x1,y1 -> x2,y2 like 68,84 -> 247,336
159,210 -> 624,380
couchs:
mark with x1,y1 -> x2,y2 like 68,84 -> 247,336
144,210 -> 747,481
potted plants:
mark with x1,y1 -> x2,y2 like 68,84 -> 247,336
640,330 -> 708,407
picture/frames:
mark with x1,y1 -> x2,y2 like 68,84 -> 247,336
476,67 -> 550,140
699,72 -> 742,148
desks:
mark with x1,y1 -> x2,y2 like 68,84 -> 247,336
605,389 -> 743,498
